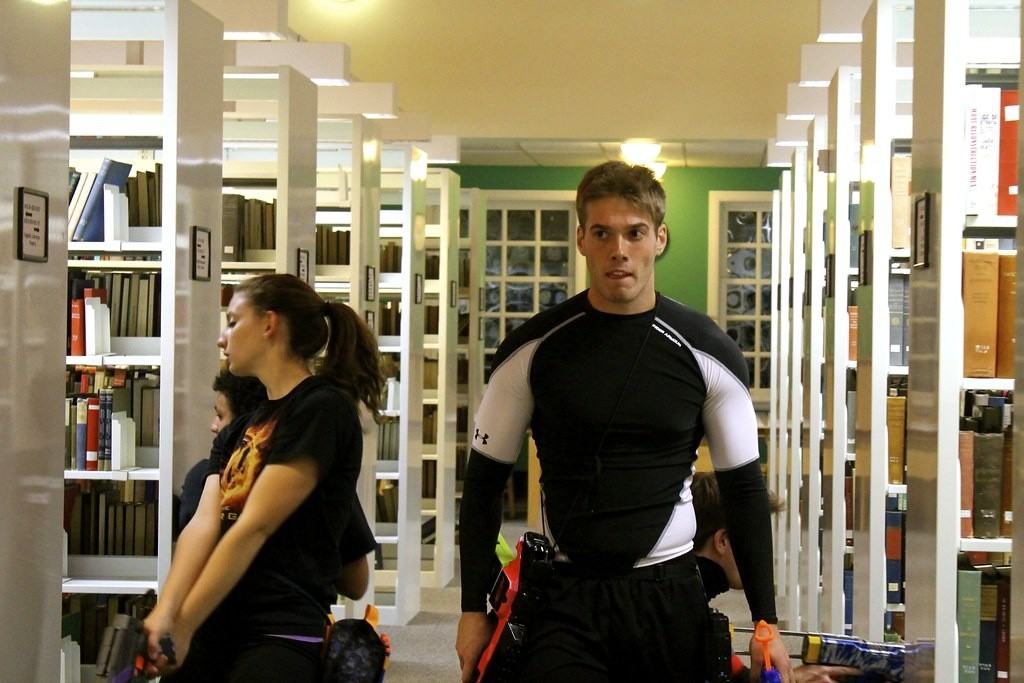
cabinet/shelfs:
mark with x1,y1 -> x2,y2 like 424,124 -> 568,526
0,0 -> 486,683
769,0 -> 1024,683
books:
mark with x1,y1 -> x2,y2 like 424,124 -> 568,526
315,224 -> 350,264
222,194 -> 276,262
59,159 -> 164,682
376,241 -> 440,523
825,83 -> 1020,683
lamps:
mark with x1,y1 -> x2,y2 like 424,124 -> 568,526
620,138 -> 667,182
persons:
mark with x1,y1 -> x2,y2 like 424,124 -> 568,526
691,471 -> 864,683
176,365 -> 377,606
142,274 -> 384,683
455,159 -> 795,683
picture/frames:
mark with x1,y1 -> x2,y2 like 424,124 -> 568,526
297,248 -> 309,286
804,270 -> 812,306
478,317 -> 485,340
366,310 -> 376,332
415,273 -> 422,304
192,225 -> 211,281
17,187 -> 49,263
451,281 -> 456,307
479,287 -> 485,312
367,266 -> 375,302
826,254 -> 832,299
913,192 -> 929,270
858,230 -> 868,286
